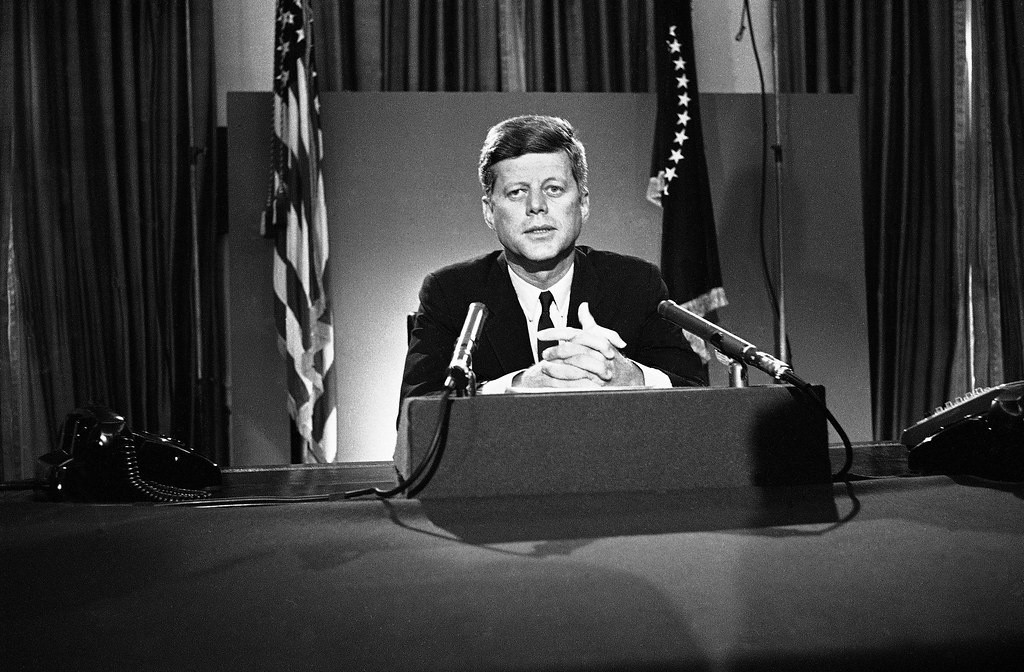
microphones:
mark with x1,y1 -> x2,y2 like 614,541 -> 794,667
449,302 -> 490,377
657,299 -> 791,383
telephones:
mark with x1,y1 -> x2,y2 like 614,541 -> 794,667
130,432 -> 223,489
909,393 -> 1024,476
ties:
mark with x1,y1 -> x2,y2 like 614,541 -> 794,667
537,291 -> 560,362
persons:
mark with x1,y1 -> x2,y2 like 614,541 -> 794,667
395,114 -> 711,434
